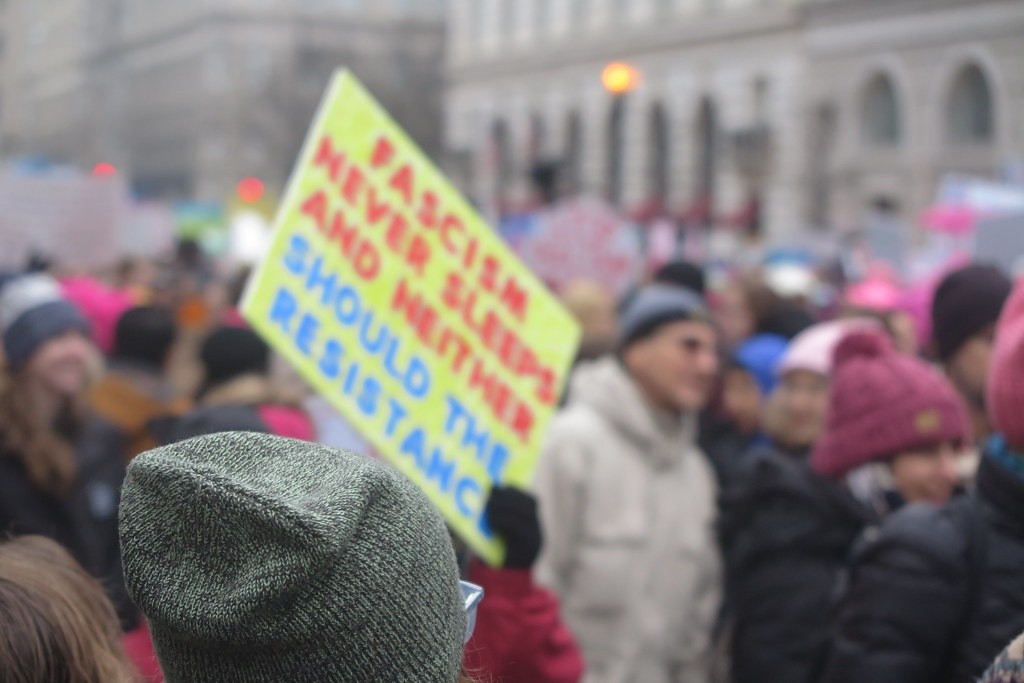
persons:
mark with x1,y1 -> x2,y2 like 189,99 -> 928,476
498,193 -> 1023,682
117,431 -> 467,683
527,261 -> 730,683
0,236 -> 369,682
445,485 -> 584,683
0,534 -> 142,683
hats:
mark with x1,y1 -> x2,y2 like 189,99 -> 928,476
614,283 -> 726,359
774,318 -> 880,376
726,330 -> 791,400
193,325 -> 270,400
808,330 -> 968,477
118,431 -> 467,683
0,272 -> 90,364
987,275 -> 1024,460
